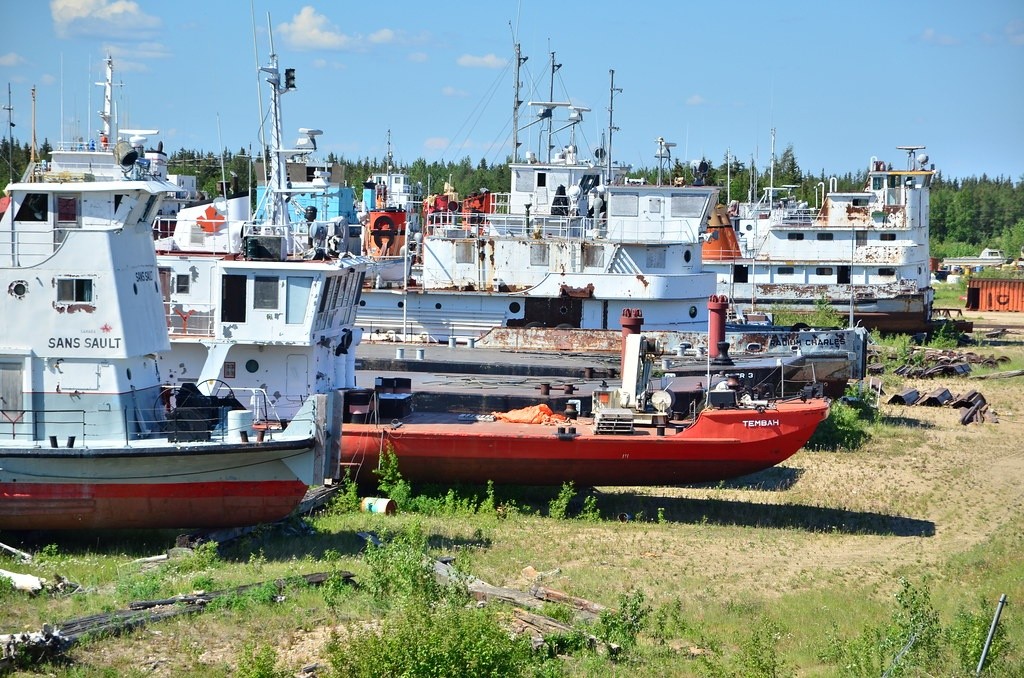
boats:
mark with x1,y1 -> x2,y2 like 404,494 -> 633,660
2,0 -> 940,542
942,247 -> 1012,271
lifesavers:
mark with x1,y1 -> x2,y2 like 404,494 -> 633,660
420,331 -> 430,342
386,330 -> 396,342
